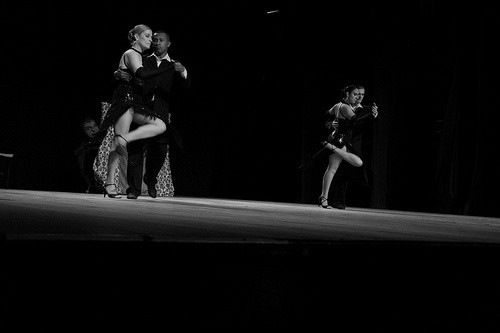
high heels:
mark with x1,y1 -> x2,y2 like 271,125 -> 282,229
103,182 -> 121,199
111,134 -> 128,156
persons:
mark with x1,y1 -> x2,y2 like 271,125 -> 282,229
319,85 -> 377,208
326,87 -> 379,210
102,24 -> 184,199
115,31 -> 188,199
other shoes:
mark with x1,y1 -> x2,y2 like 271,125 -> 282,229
144,176 -> 158,198
127,190 -> 137,199
